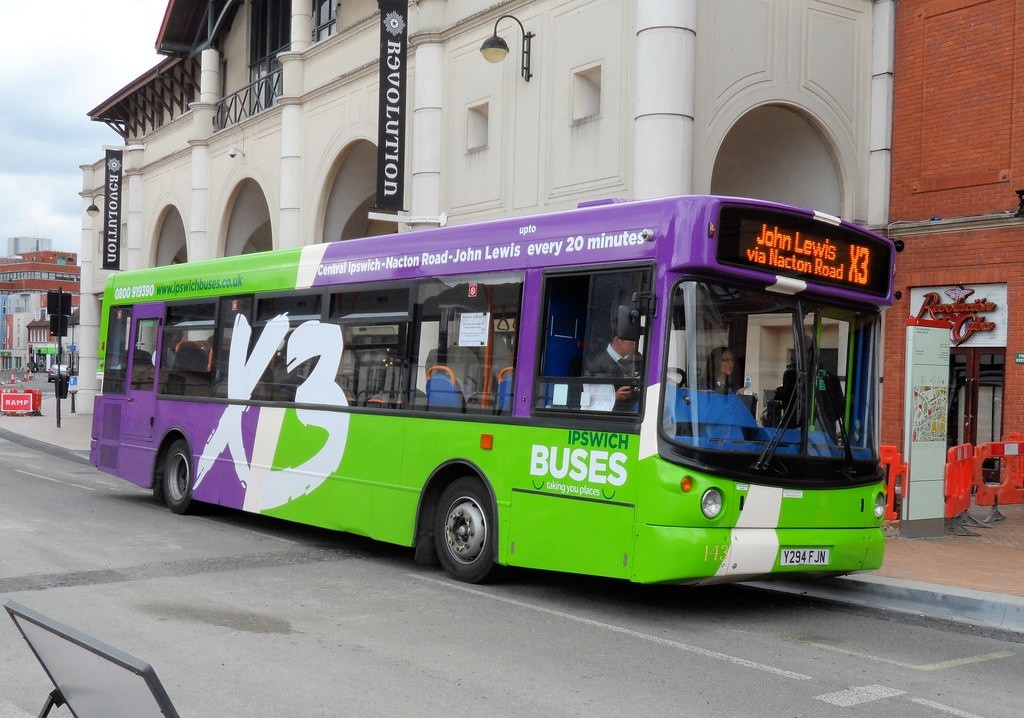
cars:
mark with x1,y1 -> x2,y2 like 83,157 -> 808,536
48,364 -> 73,383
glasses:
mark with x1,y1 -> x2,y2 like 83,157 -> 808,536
721,358 -> 732,362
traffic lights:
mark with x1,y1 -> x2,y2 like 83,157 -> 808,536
28,345 -> 32,354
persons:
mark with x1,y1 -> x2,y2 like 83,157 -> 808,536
582,320 -> 642,400
773,336 -> 844,444
152,331 -> 184,367
259,373 -> 289,401
701,346 -> 747,394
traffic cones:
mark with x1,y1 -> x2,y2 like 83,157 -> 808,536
22,372 -> 27,382
11,370 -> 15,384
28,369 -> 33,380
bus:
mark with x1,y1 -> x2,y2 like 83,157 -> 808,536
89,191 -> 905,595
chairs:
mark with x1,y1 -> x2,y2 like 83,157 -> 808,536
208,344 -> 230,376
566,354 -> 596,407
497,367 -> 513,411
121,349 -> 153,368
425,365 -> 467,408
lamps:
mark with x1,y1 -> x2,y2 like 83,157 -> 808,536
479,15 -> 536,82
86,193 -> 105,217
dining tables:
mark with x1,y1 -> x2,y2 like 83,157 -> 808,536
175,341 -> 208,370
